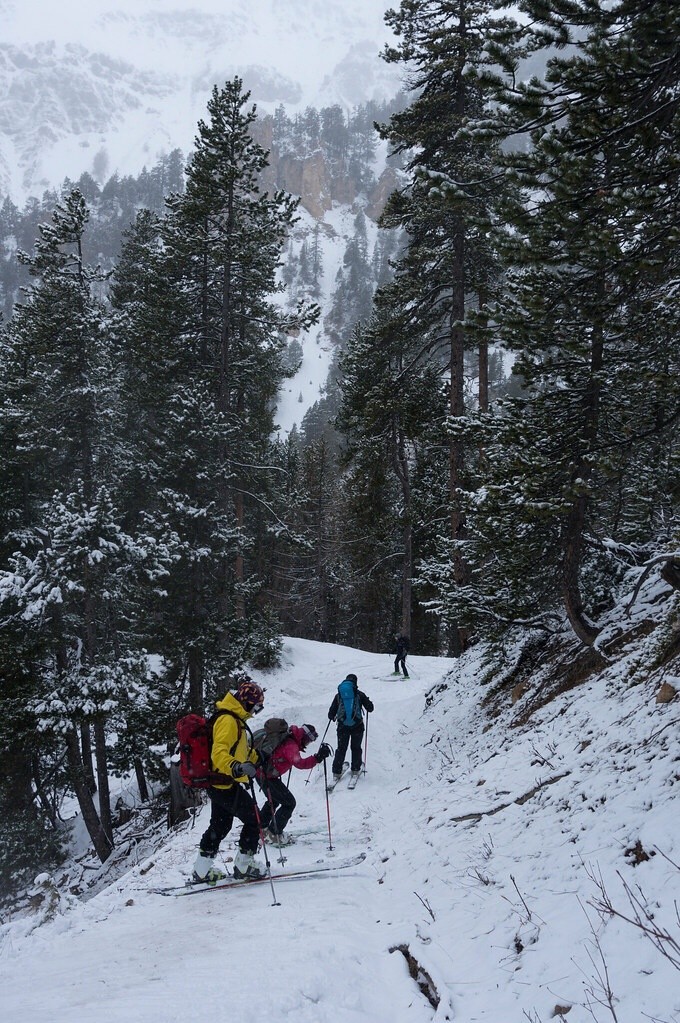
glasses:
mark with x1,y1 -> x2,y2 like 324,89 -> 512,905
253,704 -> 264,714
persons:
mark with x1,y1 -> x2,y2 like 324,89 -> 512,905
250,724 -> 331,847
388,633 -> 410,679
184,677 -> 266,884
327,675 -> 375,777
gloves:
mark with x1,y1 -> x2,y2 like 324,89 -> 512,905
313,745 -> 331,764
236,762 -> 257,778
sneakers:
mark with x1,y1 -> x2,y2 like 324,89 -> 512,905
391,672 -> 400,676
257,828 -> 295,849
191,848 -> 226,883
233,848 -> 268,882
401,675 -> 410,680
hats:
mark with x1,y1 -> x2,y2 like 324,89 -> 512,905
394,633 -> 401,639
236,681 -> 264,714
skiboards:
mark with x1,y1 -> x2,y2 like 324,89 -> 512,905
373,672 -> 421,681
223,820 -> 372,844
325,762 -> 365,790
156,852 -> 367,897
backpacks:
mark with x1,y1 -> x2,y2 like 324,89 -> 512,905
336,679 -> 364,729
244,718 -> 294,770
176,708 -> 243,791
